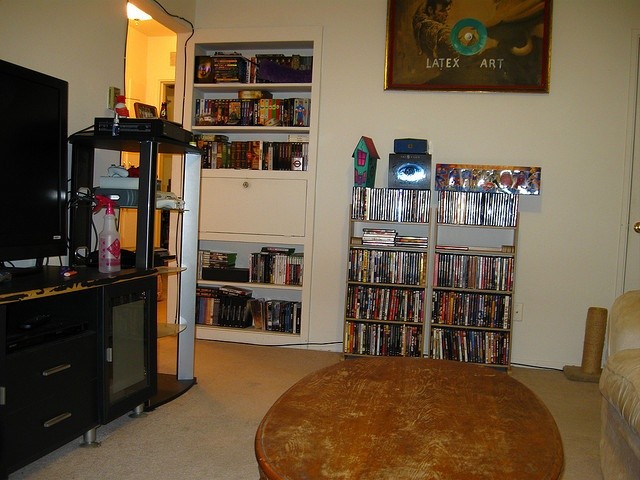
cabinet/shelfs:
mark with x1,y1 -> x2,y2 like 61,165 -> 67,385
0,265 -> 157,480
182,26 -> 323,349
343,206 -> 521,372
68,132 -> 205,412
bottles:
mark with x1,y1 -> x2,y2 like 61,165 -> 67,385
115,95 -> 128,118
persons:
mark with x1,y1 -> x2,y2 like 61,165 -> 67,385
413,0 -> 461,63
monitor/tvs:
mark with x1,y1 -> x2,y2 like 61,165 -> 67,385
0,61 -> 68,279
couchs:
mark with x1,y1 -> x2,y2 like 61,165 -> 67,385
596,290 -> 640,480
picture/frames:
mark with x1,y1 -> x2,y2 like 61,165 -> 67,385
383,0 -> 555,95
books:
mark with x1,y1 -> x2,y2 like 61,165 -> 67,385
212,50 -> 257,84
256,55 -> 311,83
352,187 -> 431,224
348,249 -> 427,285
197,249 -> 237,280
346,286 -> 425,324
438,190 -> 518,226
196,90 -> 311,127
433,254 -> 514,292
193,134 -> 309,171
196,285 -> 255,329
431,291 -> 512,330
249,298 -> 301,334
361,228 -> 428,248
434,245 -> 469,250
250,246 -> 304,288
344,321 -> 422,357
431,327 -> 510,365
